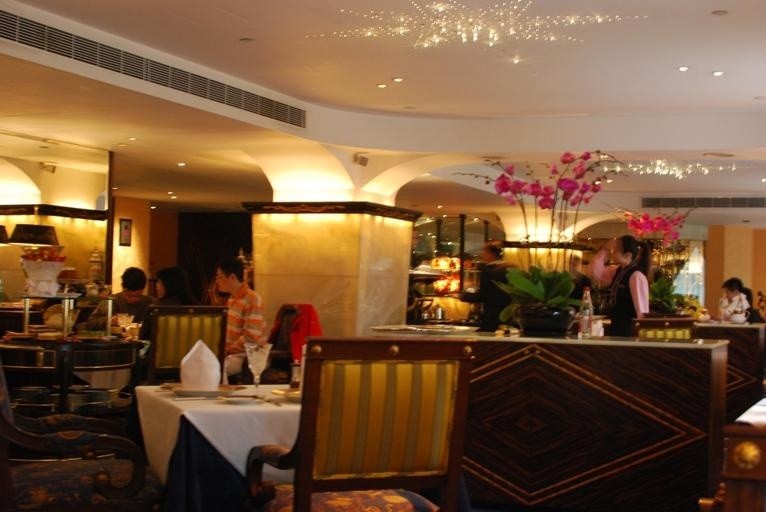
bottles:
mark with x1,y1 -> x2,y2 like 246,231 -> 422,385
577,287 -> 593,338
289,357 -> 302,388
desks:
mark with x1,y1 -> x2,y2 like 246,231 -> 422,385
695,319 -> 766,424
373,321 -> 730,512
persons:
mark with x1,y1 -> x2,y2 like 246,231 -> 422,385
89,267 -> 160,365
454,238 -> 518,335
718,280 -> 749,322
153,267 -> 191,330
605,233 -> 652,339
210,255 -> 267,385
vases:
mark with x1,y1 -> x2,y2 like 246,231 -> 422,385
20,262 -> 64,296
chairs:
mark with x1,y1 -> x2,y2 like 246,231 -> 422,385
242,302 -> 326,383
0,371 -> 165,512
246,336 -> 476,512
135,306 -> 228,384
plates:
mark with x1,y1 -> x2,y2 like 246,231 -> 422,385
172,388 -> 236,397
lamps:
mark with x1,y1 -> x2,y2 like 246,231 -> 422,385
0,225 -> 10,246
9,207 -> 59,246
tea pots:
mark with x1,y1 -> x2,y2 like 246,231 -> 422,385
723,309 -> 751,324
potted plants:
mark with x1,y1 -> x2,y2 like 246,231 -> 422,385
493,267 -> 577,338
643,278 -> 677,318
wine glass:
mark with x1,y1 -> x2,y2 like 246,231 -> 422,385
244,342 -> 273,397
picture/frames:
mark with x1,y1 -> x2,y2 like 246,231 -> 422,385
120,219 -> 132,245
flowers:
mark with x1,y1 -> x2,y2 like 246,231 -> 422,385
454,150 -> 630,274
624,208 -> 683,247
21,247 -> 66,261
